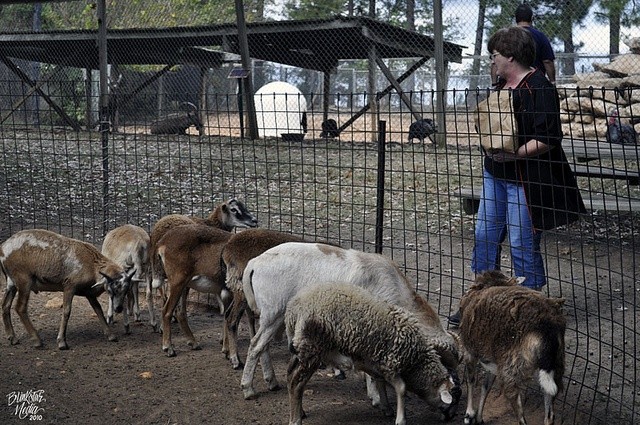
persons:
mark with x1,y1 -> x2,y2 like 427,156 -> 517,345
473,30 -> 598,293
514,3 -> 560,85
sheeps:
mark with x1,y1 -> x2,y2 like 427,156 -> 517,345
0,228 -> 144,351
218,222 -> 351,399
282,279 -> 457,423
147,199 -> 259,330
242,240 -> 463,423
149,220 -> 244,370
102,220 -> 160,336
460,268 -> 570,423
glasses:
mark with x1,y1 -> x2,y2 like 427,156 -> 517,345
490,53 -> 500,59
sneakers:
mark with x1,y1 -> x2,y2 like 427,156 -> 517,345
447,310 -> 464,325
